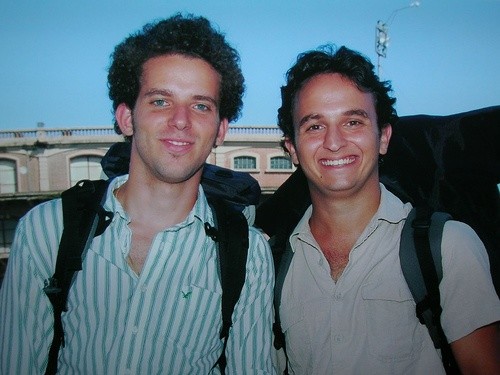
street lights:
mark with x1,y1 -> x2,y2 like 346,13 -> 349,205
375,2 -> 422,81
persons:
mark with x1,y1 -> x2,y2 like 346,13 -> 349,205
0,10 -> 277,375
267,41 -> 500,375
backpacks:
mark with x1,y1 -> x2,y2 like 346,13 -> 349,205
43,142 -> 262,375
